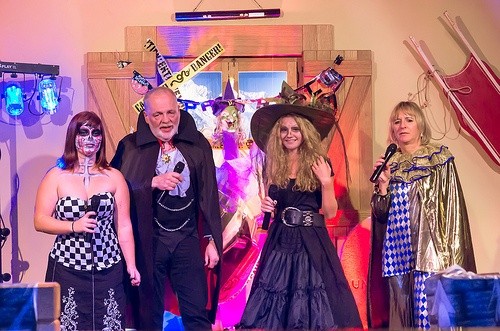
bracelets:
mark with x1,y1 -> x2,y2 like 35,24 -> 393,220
72,221 -> 76,232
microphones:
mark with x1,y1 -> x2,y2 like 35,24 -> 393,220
369,143 -> 397,183
85,195 -> 101,242
156,161 -> 186,204
262,184 -> 278,230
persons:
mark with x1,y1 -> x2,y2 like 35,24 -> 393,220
110,87 -> 223,331
366,100 -> 477,331
235,104 -> 364,331
34,111 -> 141,331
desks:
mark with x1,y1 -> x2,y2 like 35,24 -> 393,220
425,272 -> 500,331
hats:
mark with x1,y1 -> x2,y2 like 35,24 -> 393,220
251,94 -> 336,154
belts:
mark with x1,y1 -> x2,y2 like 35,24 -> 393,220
276,207 -> 325,227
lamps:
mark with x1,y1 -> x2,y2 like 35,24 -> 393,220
2,72 -> 26,118
36,72 -> 63,117
